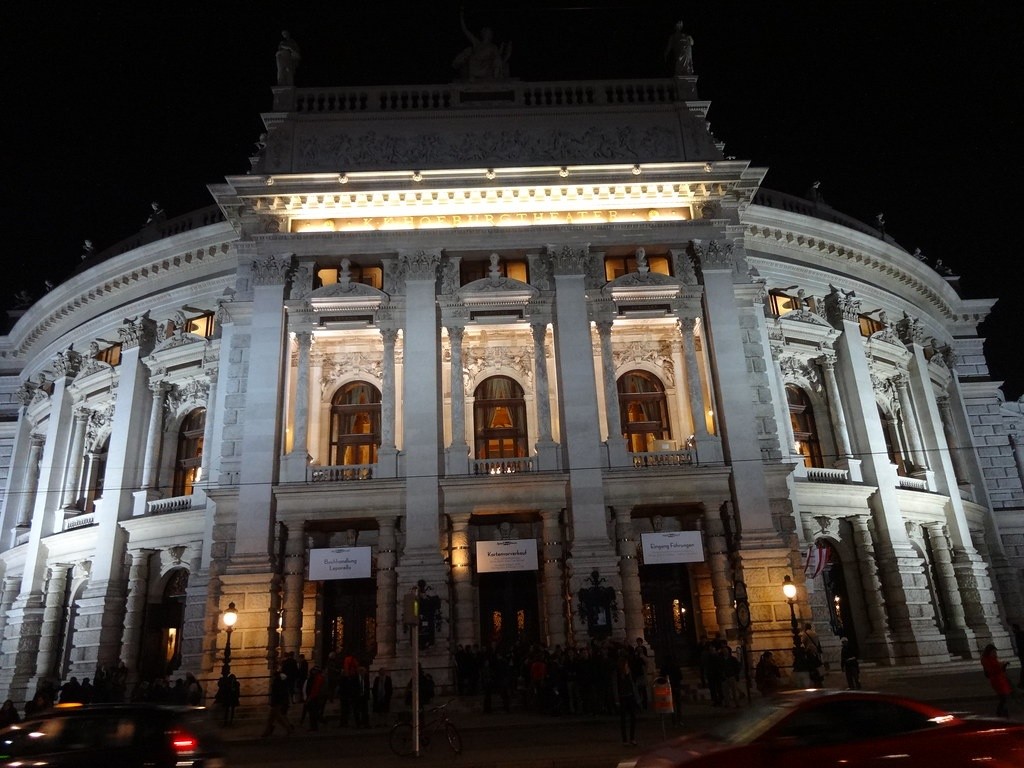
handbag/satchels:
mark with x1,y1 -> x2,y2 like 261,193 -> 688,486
817,645 -> 823,654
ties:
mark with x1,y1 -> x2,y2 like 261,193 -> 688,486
379,676 -> 385,694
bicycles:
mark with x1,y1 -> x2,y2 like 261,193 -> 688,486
388,698 -> 463,757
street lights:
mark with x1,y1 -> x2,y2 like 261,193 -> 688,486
782,575 -> 811,689
221,602 -> 238,678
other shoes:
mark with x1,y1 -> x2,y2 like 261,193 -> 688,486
305,728 -> 318,734
623,739 -> 638,748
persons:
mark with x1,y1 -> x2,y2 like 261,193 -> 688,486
913,247 -> 953,278
170,672 -> 200,707
0,699 -> 20,728
36,375 -> 45,389
488,253 -> 500,271
841,637 -> 861,690
405,661 -> 435,724
635,247 -> 647,265
25,677 -> 95,714
80,341 -> 100,368
796,290 -> 808,305
456,635 -> 649,747
659,653 -> 687,728
879,312 -> 891,327
982,644 -> 1014,718
932,339 -> 944,355
802,623 -> 822,682
174,311 -> 187,328
697,632 -> 743,707
221,674 -> 240,728
756,651 -> 779,698
339,258 -> 352,276
261,652 -> 393,738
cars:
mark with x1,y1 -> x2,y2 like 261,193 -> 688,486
0,703 -> 228,768
618,689 -> 1024,768
